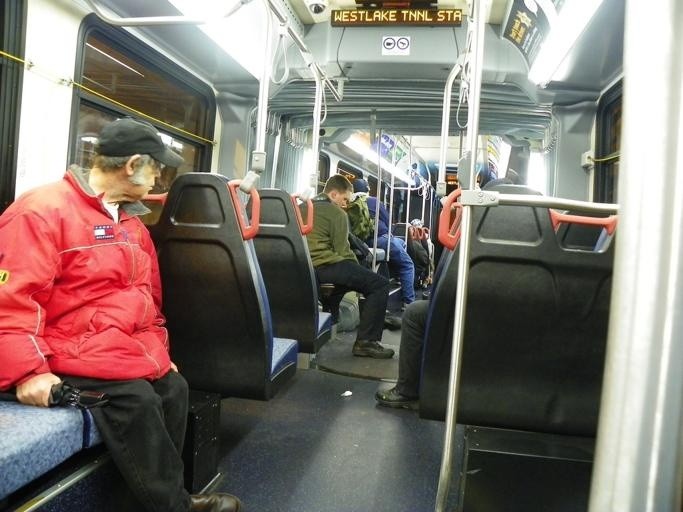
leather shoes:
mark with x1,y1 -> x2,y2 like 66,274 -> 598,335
189,493 -> 242,511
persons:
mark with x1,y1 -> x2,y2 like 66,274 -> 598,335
0,114 -> 246,512
297,175 -> 395,360
373,299 -> 430,412
408,217 -> 433,288
353,179 -> 417,312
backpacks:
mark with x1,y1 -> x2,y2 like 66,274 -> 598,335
347,195 -> 374,241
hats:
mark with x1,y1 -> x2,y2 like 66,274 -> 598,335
353,179 -> 371,192
95,117 -> 185,168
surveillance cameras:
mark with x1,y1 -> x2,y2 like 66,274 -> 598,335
311,5 -> 325,14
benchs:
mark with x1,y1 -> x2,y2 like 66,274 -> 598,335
392,223 -> 434,290
144,171 -> 298,503
417,184 -> 618,441
1,397 -> 114,510
429,177 -> 617,303
241,189 -> 333,355
362,247 -> 386,275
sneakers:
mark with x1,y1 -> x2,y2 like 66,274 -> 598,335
319,295 -> 339,325
352,340 -> 394,360
401,301 -> 408,311
375,386 -> 420,408
